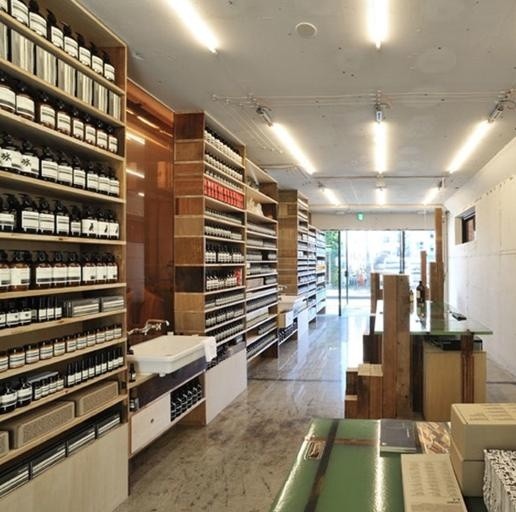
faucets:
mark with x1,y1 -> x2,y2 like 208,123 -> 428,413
138,319 -> 171,336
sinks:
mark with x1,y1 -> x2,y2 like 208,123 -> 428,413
128,334 -> 212,375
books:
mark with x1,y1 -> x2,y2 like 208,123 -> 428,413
377,419 -> 423,454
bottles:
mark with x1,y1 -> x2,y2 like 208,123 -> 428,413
0,347 -> 124,414
128,364 -> 139,414
205,288 -> 244,344
171,381 -> 202,422
409,289 -> 414,304
417,281 -> 426,303
0,296 -> 62,329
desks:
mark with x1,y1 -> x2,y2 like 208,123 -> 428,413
270,416 -> 489,512
374,299 -> 493,410
